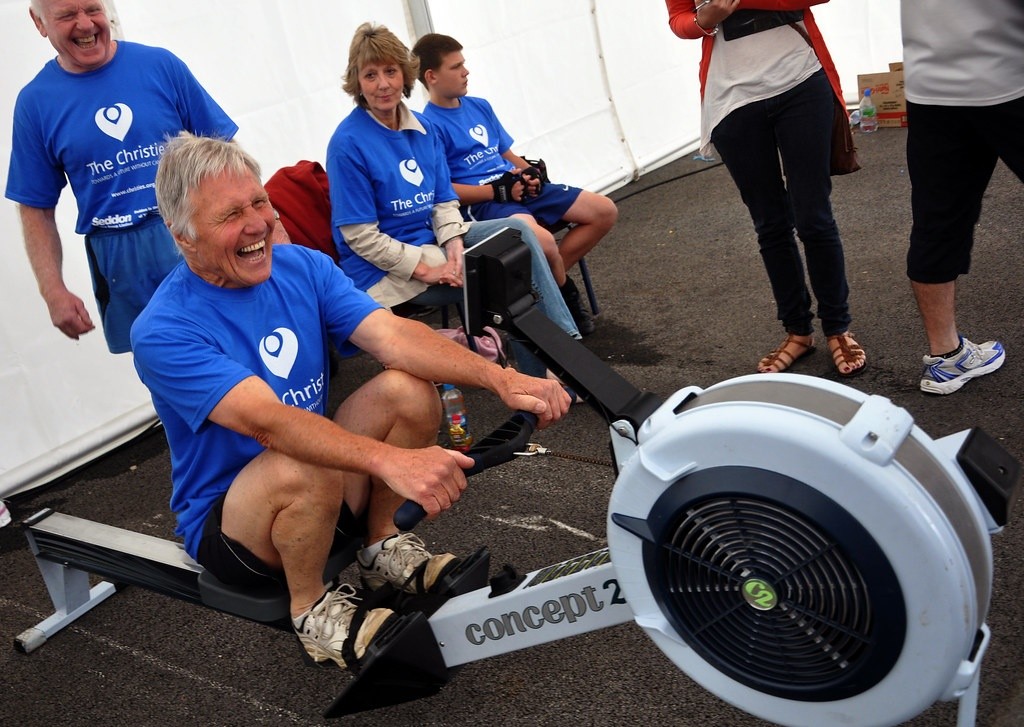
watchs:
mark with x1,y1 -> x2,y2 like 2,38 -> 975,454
273,208 -> 280,220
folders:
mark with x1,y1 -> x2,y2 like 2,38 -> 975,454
723,8 -> 804,41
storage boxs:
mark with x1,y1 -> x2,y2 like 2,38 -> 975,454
857,62 -> 907,127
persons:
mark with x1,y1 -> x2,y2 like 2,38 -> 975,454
664,0 -> 870,377
411,33 -> 619,334
5,0 -> 291,353
900,0 -> 1024,395
129,129 -> 572,668
327,22 -> 583,404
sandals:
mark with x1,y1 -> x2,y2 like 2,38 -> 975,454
827,334 -> 868,380
756,334 -> 815,373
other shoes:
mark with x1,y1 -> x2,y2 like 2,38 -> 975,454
561,278 -> 596,335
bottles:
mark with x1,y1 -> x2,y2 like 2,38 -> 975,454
441,384 -> 473,447
858,89 -> 879,133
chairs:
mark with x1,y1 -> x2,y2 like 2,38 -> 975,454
262,162 -> 449,328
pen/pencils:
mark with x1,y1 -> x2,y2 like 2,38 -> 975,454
692,0 -> 710,13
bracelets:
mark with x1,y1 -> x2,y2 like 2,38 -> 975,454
693,15 -> 719,37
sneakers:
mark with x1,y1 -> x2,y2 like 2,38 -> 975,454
918,336 -> 1006,395
357,533 -> 461,594
292,583 -> 394,670
